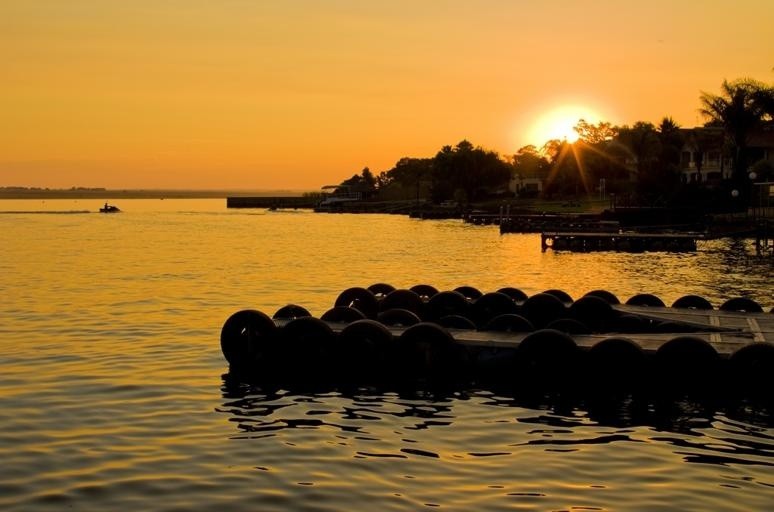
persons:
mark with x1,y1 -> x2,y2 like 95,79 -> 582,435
605,188 -> 634,210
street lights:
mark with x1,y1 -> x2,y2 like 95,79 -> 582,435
731,190 -> 738,216
748,172 -> 757,216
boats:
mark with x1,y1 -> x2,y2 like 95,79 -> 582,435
468,212 -> 696,253
221,283 -> 774,403
100,206 -> 119,212
